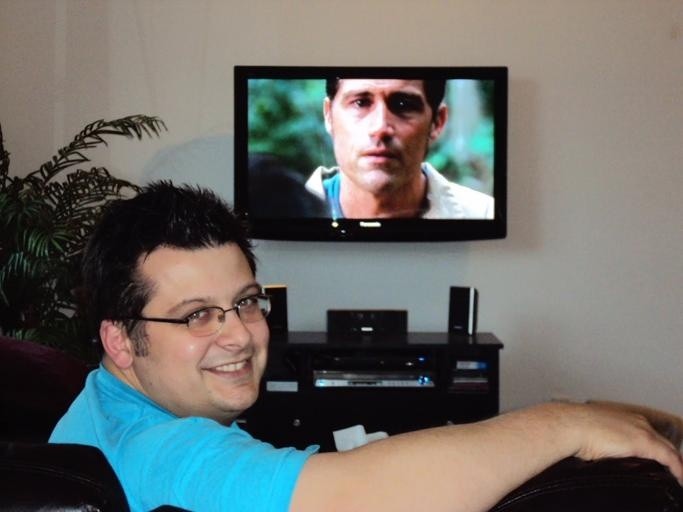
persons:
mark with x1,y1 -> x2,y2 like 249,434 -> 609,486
45,181 -> 683,512
303,78 -> 495,219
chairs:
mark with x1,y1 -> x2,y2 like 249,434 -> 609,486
487,457 -> 683,512
0,444 -> 194,512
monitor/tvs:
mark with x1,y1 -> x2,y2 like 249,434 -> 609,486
233,65 -> 508,243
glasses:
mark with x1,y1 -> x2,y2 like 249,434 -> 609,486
108,294 -> 275,338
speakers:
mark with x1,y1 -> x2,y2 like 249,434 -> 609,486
327,309 -> 407,337
448,285 -> 479,336
262,285 -> 289,336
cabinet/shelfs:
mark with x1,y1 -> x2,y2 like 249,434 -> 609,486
236,331 -> 505,454
